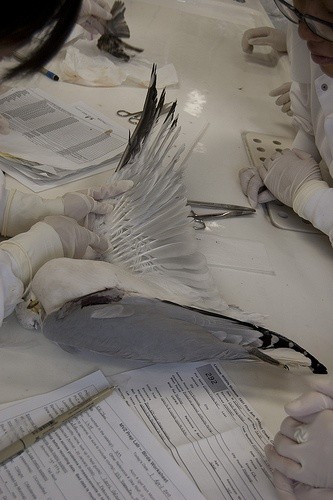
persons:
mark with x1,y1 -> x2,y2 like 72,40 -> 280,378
239,0 -> 333,245
0,0 -> 134,317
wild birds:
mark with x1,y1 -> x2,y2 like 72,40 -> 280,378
16,64 -> 328,376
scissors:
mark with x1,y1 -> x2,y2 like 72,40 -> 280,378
117,101 -> 176,125
185,209 -> 253,230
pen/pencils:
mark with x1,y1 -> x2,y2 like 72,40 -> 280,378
9,52 -> 59,82
1,378 -> 119,464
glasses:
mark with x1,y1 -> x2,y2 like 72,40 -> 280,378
274,0 -> 333,43
